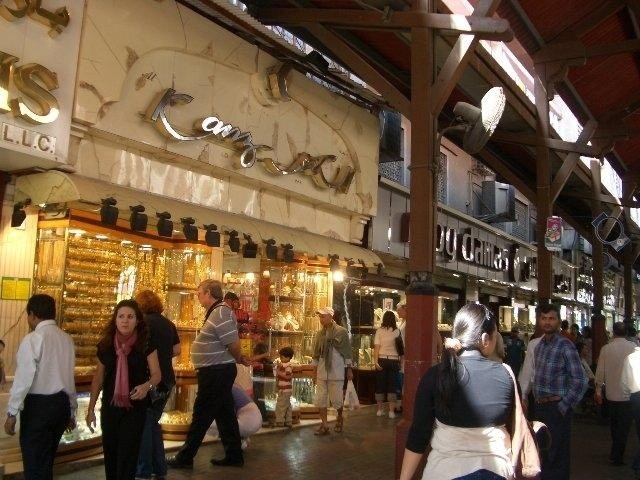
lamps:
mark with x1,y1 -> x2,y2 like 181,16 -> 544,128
243,233 -> 257,258
129,205 -> 148,233
225,229 -> 238,252
181,217 -> 199,242
156,211 -> 173,237
329,254 -> 344,282
10,198 -> 32,228
262,238 -> 278,259
281,244 -> 295,261
261,264 -> 271,280
101,198 -> 119,226
205,224 -> 219,248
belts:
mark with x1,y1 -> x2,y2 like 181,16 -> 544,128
537,394 -> 561,407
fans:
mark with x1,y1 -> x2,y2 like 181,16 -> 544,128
438,85 -> 508,163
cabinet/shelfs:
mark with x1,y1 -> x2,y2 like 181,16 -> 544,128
291,260 -> 332,422
348,271 -> 408,406
261,260 -> 307,424
158,234 -> 225,439
33,209 -> 157,466
225,252 -> 260,364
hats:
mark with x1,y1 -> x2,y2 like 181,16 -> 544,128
315,307 -> 334,316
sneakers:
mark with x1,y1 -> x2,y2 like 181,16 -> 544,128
272,422 -> 284,427
377,410 -> 386,417
284,421 -> 292,426
389,411 -> 399,419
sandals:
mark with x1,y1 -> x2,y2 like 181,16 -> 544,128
334,418 -> 343,433
314,425 -> 329,435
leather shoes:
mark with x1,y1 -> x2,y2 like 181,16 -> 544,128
165,455 -> 193,469
211,456 -> 244,466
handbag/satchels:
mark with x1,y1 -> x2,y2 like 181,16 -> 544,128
502,363 -> 552,480
395,330 -> 404,356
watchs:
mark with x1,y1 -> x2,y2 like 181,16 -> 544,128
7,413 -> 15,417
145,382 -> 153,391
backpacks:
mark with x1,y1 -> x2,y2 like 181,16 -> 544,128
559,338 -> 596,415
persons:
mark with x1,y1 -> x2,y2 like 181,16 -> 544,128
274,346 -> 294,427
394,299 -> 406,414
164,278 -> 255,469
593,321 -> 640,466
223,290 -> 250,338
84,299 -> 163,479
560,320 -> 576,343
252,342 -> 273,429
372,311 -> 404,418
2,293 -> 80,480
569,323 -> 584,353
579,326 -> 593,368
312,306 -> 355,435
134,290 -> 182,479
504,326 -> 526,396
572,342 -> 595,420
397,303 -> 524,480
521,303 -> 589,479
